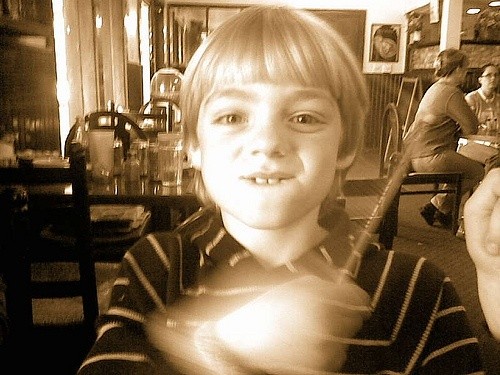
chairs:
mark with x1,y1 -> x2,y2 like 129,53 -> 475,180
379,102 -> 462,237
0,66 -> 184,327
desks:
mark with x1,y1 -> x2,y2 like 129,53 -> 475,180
457,135 -> 500,164
26,169 -> 199,290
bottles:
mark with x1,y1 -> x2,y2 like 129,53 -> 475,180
74,116 -> 87,168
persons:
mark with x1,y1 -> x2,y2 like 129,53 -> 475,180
76,4 -> 500,375
410,48 -> 500,241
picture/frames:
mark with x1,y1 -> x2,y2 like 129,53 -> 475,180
369,23 -> 401,62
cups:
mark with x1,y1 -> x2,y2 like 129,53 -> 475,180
87,128 -> 185,188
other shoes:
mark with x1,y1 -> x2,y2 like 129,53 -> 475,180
418,203 -> 436,227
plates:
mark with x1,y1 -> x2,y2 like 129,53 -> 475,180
32,159 -> 66,169
